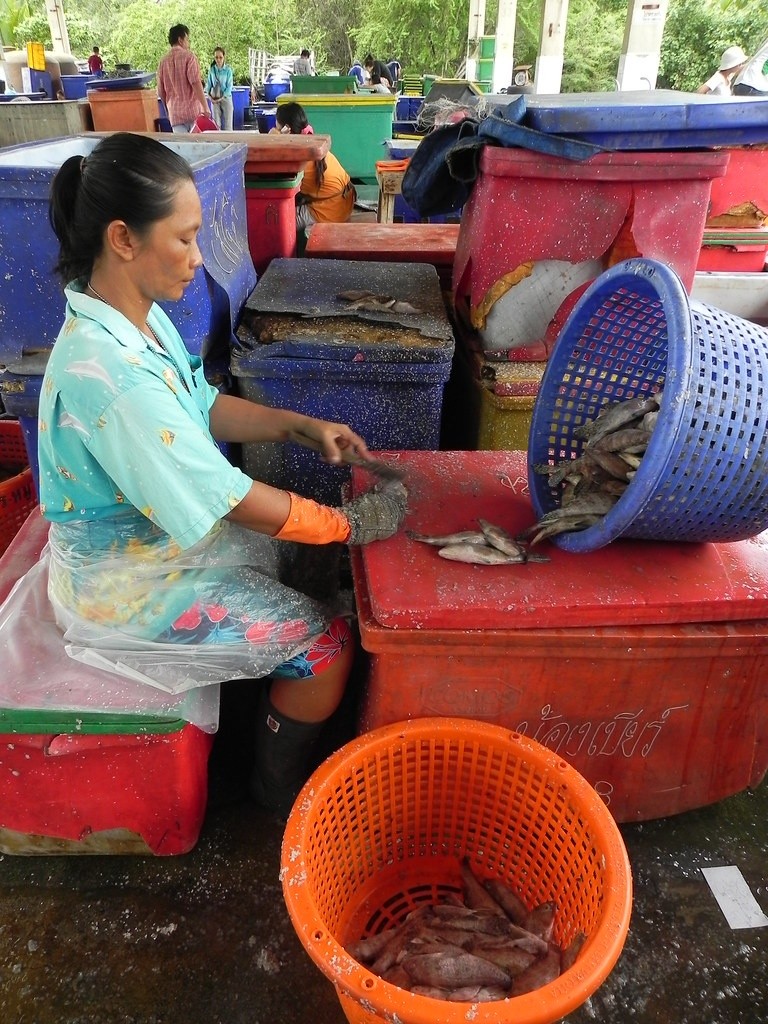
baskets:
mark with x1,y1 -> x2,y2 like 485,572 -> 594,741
280,717 -> 632,1024
526,256 -> 768,555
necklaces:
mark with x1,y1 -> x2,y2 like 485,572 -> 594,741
85,279 -> 189,392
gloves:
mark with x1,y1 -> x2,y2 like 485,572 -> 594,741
267,491 -> 401,545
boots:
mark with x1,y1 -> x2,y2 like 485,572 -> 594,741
245,694 -> 333,819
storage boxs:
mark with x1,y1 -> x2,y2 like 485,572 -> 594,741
0,68 -> 768,857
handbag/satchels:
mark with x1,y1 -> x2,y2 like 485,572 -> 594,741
208,85 -> 221,99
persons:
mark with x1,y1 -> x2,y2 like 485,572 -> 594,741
56,90 -> 65,100
364,54 -> 393,88
207,47 -> 233,131
157,24 -> 211,134
268,103 -> 358,233
36,134 -> 407,812
88,47 -> 103,77
697,46 -> 748,96
347,60 -> 365,85
293,50 -> 311,76
363,75 -> 391,94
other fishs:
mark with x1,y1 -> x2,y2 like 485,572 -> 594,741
438,543 -> 551,565
514,390 -> 768,546
336,289 -> 375,300
348,855 -> 586,1003
344,296 -> 396,314
405,530 -> 489,546
392,301 -> 428,314
477,518 -> 521,557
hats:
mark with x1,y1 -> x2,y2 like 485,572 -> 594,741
717,46 -> 750,71
